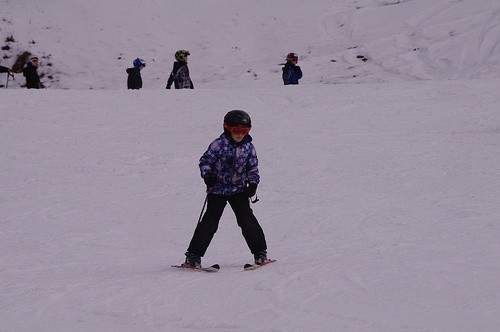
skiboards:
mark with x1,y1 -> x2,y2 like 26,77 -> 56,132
170,259 -> 276,273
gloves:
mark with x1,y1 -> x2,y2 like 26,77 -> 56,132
242,183 -> 257,198
204,173 -> 217,187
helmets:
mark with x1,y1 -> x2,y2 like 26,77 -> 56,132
223,110 -> 251,137
133,58 -> 146,69
175,49 -> 191,61
286,52 -> 298,64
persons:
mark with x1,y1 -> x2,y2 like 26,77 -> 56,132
23,56 -> 45,89
165,49 -> 194,89
282,53 -> 303,85
185,110 -> 269,267
126,57 -> 146,91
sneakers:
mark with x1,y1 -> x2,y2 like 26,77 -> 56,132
185,251 -> 202,268
254,248 -> 269,264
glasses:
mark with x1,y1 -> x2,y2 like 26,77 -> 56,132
224,124 -> 250,135
